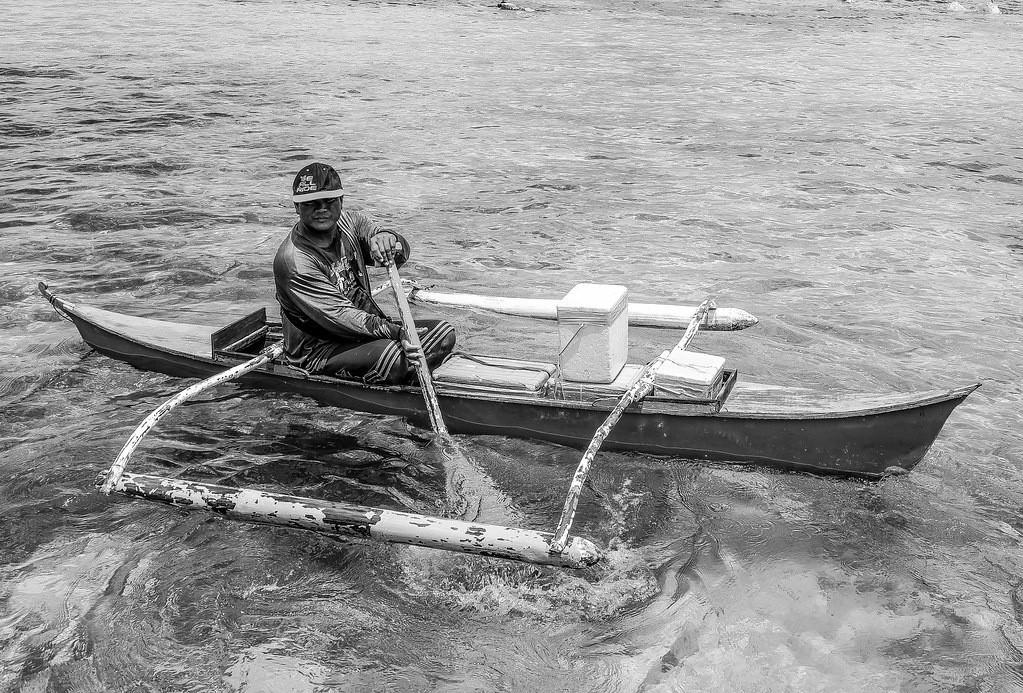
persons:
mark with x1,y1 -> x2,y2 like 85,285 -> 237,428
273,163 -> 456,387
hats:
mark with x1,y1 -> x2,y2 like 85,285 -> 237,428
292,163 -> 345,203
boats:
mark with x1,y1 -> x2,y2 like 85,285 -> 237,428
38,280 -> 982,485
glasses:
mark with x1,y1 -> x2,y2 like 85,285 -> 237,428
301,198 -> 338,206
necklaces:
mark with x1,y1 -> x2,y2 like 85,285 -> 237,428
296,223 -> 344,293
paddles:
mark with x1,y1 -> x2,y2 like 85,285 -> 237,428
386,262 -> 524,530
